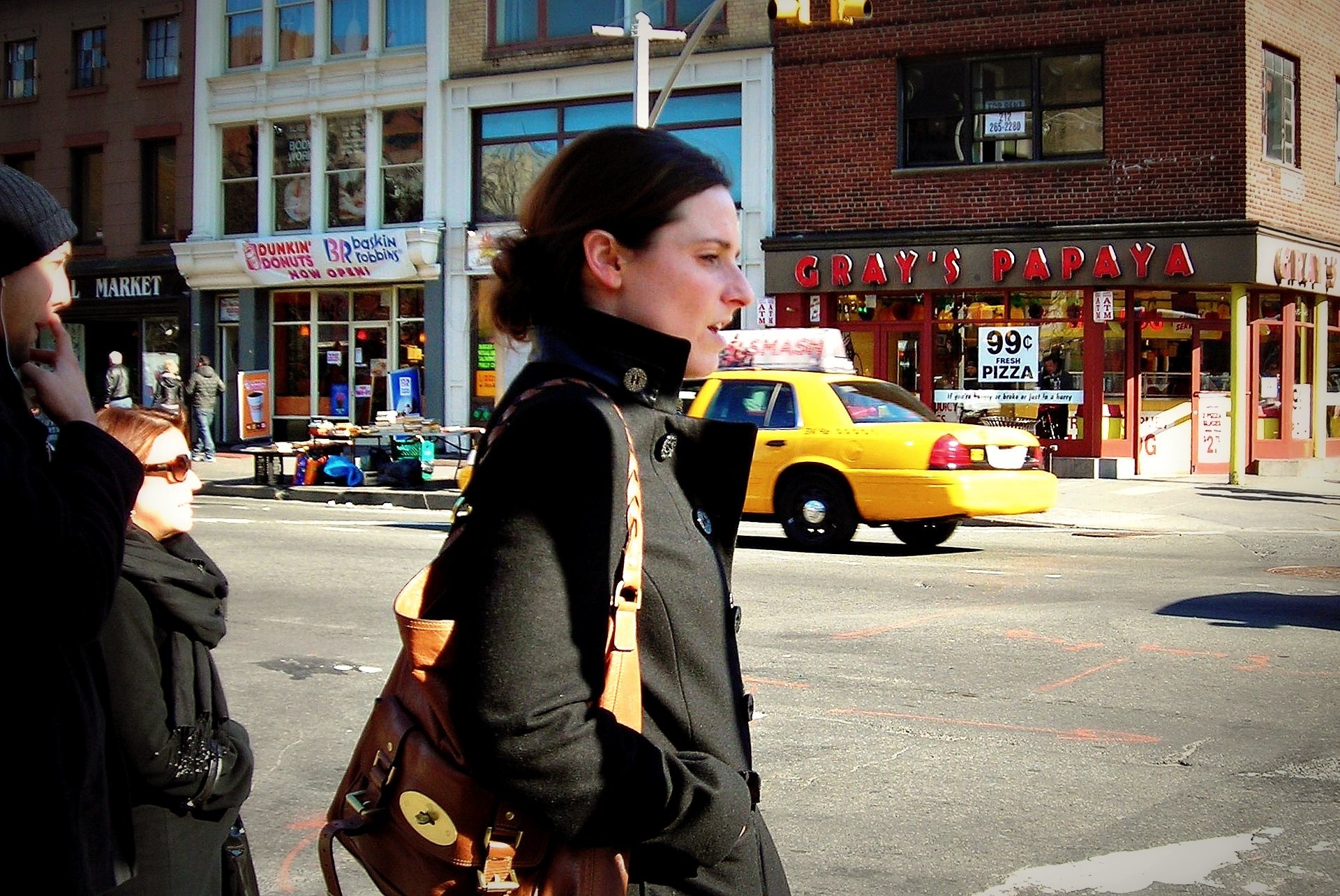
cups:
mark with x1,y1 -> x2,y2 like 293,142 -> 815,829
246,391 -> 263,423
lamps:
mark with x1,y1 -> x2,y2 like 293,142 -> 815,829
466,223 -> 478,237
357,329 -> 368,341
299,326 -> 310,337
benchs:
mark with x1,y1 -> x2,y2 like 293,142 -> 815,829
273,396 -> 333,415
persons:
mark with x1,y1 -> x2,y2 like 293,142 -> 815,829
965,353 -> 1073,438
103,351 -> 133,409
1,162 -> 145,896
25,389 -> 59,463
418,126 -> 795,896
96,404 -> 260,896
185,355 -> 226,463
150,359 -> 184,414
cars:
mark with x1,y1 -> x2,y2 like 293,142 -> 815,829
679,368 -> 1058,552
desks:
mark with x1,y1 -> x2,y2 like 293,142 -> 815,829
317,427 -> 486,488
232,442 -> 297,485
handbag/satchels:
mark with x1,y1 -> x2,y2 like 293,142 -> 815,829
318,378 -> 644,896
221,815 -> 260,896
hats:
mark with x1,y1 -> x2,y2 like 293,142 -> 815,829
0,161 -> 79,278
109,350 -> 123,365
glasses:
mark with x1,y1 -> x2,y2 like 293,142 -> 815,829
141,454 -> 193,481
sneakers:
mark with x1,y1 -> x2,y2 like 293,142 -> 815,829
192,455 -> 215,462
